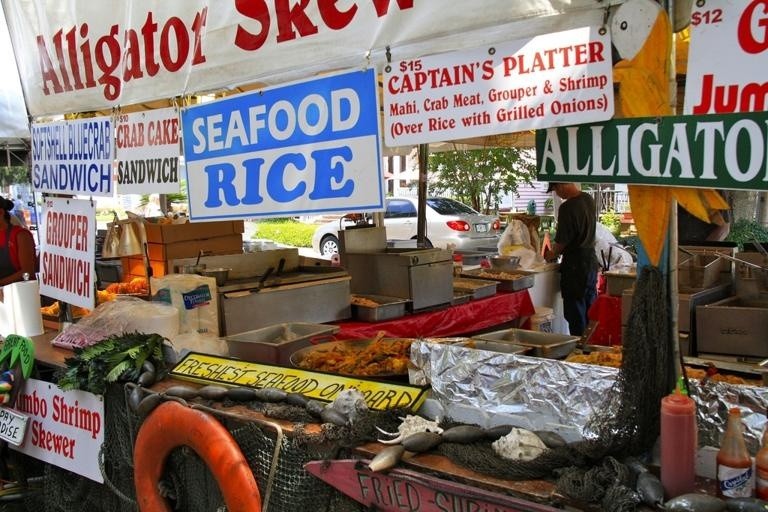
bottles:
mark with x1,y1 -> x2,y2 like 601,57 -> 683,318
716,408 -> 768,500
658,375 -> 698,500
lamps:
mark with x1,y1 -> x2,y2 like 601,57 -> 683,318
102,211 -> 153,258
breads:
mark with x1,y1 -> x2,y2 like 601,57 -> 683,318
565,349 -> 748,385
40,276 -> 148,319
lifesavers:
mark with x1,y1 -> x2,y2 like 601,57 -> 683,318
134,401 -> 263,512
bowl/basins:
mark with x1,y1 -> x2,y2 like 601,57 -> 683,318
198,266 -> 232,287
488,254 -> 521,268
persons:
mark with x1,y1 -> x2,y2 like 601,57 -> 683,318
0,194 -> 38,287
677,188 -> 730,244
542,181 -> 601,338
6,194 -> 36,226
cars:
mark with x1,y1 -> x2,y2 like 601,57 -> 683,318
312,195 -> 502,258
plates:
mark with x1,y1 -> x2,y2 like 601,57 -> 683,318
289,337 -> 423,379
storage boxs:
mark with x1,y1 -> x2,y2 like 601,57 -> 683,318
122,219 -> 245,282
622,241 -> 768,357
